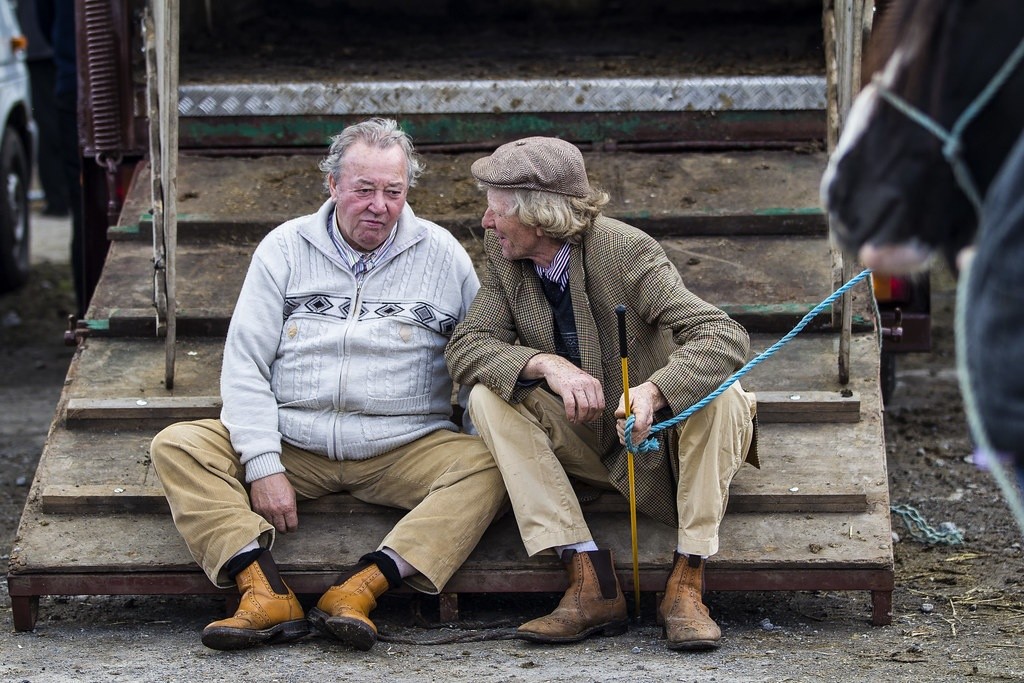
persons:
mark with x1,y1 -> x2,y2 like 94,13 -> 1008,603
444,134 -> 760,645
151,118 -> 505,649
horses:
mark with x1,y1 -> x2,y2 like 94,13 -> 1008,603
819,0 -> 1023,487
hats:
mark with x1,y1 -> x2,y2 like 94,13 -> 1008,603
470,136 -> 590,198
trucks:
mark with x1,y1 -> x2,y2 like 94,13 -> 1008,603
6,1 -> 909,632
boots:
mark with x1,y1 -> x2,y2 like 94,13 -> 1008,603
202,547 -> 305,651
660,550 -> 722,651
517,547 -> 627,643
307,551 -> 402,651
